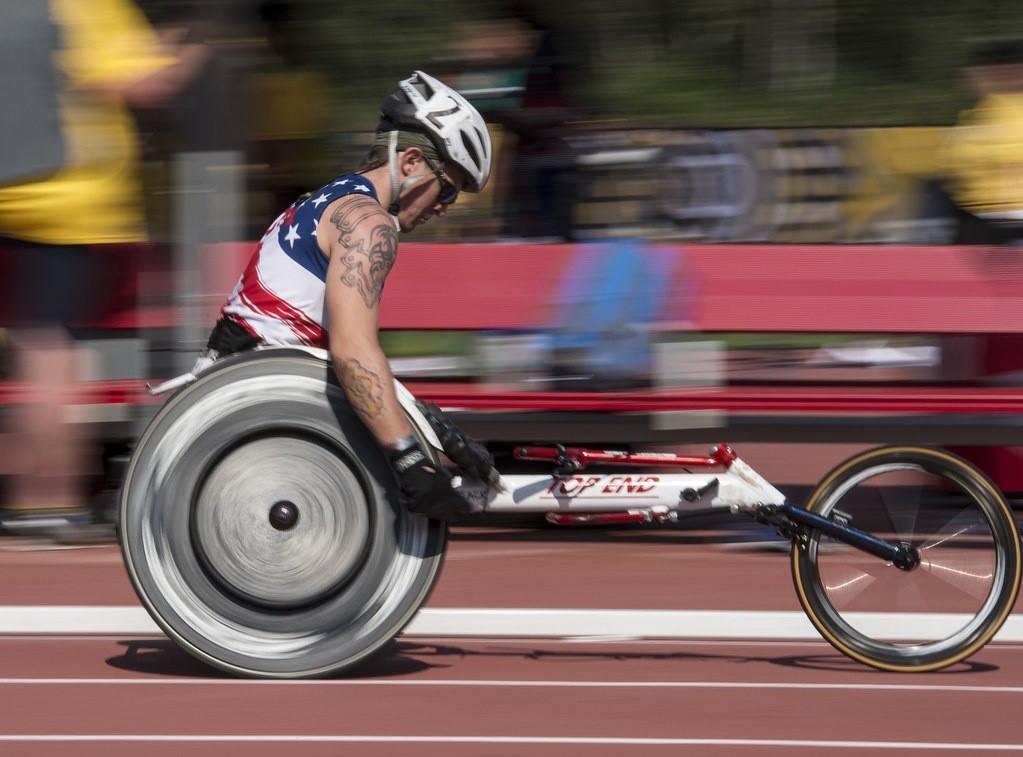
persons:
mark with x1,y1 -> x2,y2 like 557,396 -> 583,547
0,0 -> 214,537
197,69 -> 502,517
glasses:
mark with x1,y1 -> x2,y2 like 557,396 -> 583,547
396,148 -> 458,206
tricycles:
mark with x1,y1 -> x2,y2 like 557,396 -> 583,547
116,345 -> 1023,680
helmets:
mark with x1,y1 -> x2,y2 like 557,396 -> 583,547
381,70 -> 492,193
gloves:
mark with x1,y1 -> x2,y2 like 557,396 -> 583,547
389,439 -> 469,521
